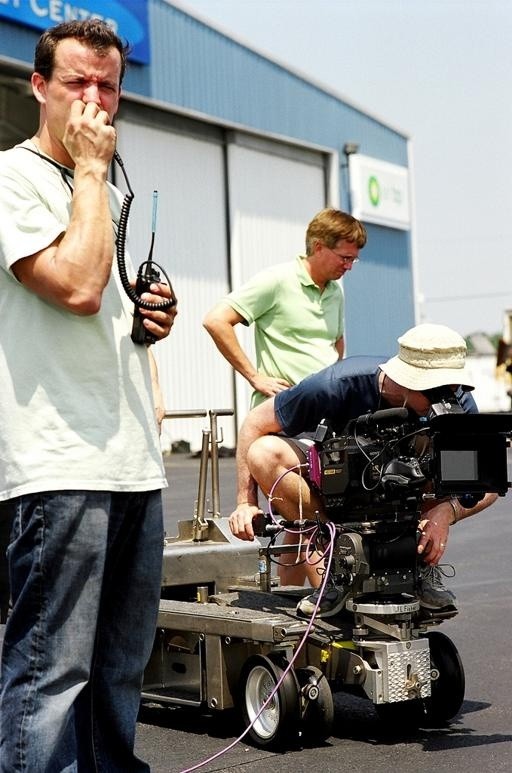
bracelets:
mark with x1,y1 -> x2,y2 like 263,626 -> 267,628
451,498 -> 461,524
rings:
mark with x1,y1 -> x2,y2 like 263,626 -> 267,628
439,543 -> 445,547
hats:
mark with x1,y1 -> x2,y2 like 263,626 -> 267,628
378,325 -> 475,392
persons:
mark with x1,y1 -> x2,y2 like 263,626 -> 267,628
0,16 -> 180,773
224,323 -> 512,569
198,205 -> 369,594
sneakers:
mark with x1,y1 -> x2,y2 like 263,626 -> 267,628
415,562 -> 458,612
297,558 -> 352,618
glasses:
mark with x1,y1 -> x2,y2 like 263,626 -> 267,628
327,246 -> 360,263
